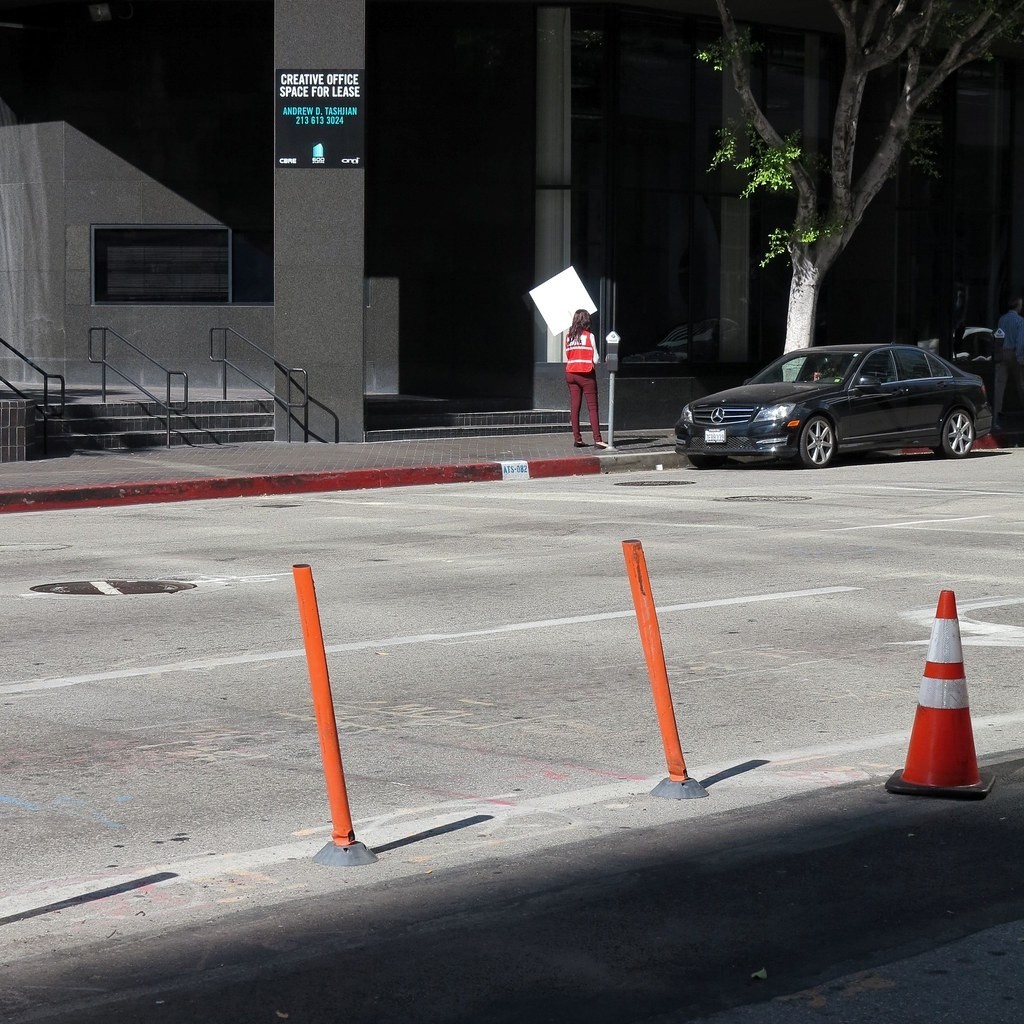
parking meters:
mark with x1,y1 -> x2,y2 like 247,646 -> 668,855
606,331 -> 620,451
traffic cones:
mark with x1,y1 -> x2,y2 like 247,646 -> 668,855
884,589 -> 996,798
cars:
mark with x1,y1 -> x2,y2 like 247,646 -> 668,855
656,318 -> 740,352
917,327 -> 994,359
674,344 -> 993,469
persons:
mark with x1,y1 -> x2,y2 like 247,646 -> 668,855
991,294 -> 1024,430
564,309 -> 608,449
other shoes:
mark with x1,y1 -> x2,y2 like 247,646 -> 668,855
573,441 -> 590,447
595,441 -> 616,449
